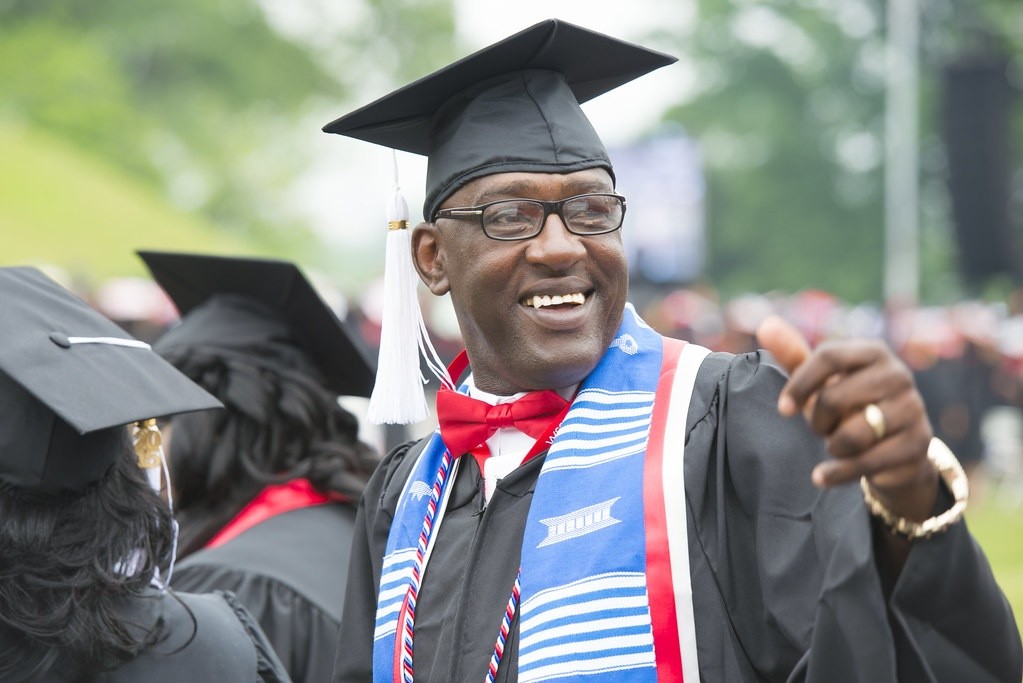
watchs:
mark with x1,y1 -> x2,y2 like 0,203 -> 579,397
859,436 -> 971,540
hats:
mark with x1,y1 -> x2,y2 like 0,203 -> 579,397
321,18 -> 680,221
0,264 -> 225,590
137,248 -> 376,439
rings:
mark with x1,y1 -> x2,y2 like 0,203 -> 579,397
864,402 -> 885,438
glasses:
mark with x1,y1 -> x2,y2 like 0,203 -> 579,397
434,191 -> 629,242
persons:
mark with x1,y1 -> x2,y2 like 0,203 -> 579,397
320,20 -> 1023,683
137,251 -> 384,683
0,265 -> 292,683
655,289 -> 1023,465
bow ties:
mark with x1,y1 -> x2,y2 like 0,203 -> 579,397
436,349 -> 569,460
